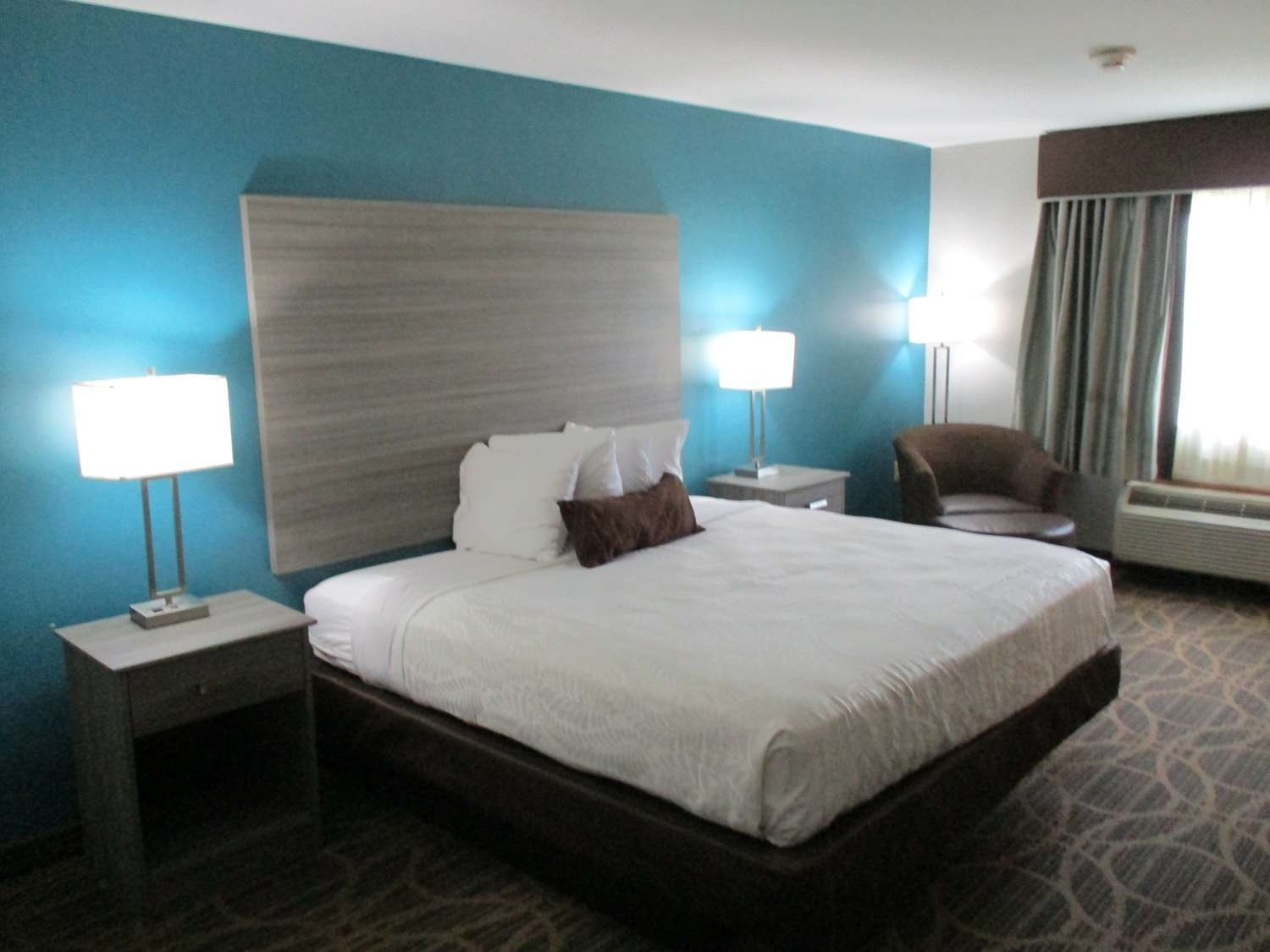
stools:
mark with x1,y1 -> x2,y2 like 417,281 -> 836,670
930,512 -> 1076,550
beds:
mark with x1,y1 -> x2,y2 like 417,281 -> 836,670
237,193 -> 1120,952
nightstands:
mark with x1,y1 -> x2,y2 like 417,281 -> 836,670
55,588 -> 320,891
707,462 -> 851,514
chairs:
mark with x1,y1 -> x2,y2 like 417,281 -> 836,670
892,422 -> 1070,525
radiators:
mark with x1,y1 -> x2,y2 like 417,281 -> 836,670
1111,479 -> 1270,584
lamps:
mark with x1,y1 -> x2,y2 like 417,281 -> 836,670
907,294 -> 957,424
70,368 -> 234,630
716,326 -> 795,478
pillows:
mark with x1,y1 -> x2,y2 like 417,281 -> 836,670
452,441 -> 577,561
486,427 -> 625,500
562,418 -> 690,492
557,471 -> 706,570
